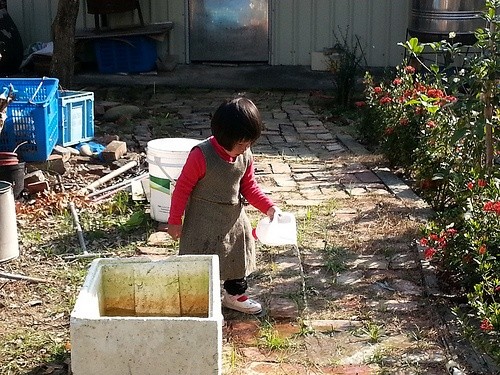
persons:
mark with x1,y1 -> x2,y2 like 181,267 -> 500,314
168,95 -> 281,315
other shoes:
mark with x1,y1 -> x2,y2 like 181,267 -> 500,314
223,291 -> 263,314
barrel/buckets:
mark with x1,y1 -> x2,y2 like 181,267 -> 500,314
0,152 -> 25,200
0,181 -> 19,262
146,138 -> 202,223
407,0 -> 487,35
